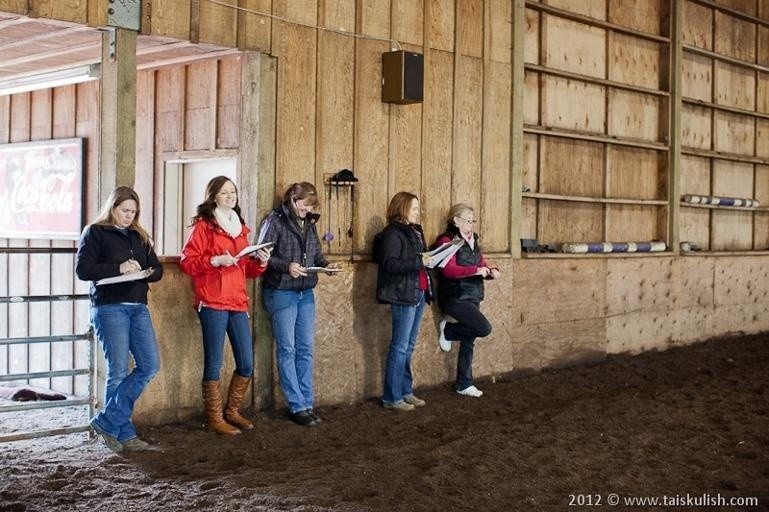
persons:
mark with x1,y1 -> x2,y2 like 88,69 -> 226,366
75,186 -> 164,452
367,191 -> 435,412
427,201 -> 502,397
178,176 -> 274,434
249,181 -> 345,427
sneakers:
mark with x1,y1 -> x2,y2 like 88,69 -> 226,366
404,395 -> 425,408
383,401 -> 414,411
439,320 -> 452,352
456,385 -> 482,397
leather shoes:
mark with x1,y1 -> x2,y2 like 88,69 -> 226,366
307,409 -> 321,424
90,421 -> 122,452
118,438 -> 149,451
290,411 -> 315,426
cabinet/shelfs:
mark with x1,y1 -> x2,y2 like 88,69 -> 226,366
674,1 -> 769,257
520,1 -> 674,259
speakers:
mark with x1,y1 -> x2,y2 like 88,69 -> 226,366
381,50 -> 423,105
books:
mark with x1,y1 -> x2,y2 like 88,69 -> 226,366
304,265 -> 344,274
235,240 -> 273,262
418,238 -> 465,270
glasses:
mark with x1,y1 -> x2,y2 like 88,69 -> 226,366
456,216 -> 477,224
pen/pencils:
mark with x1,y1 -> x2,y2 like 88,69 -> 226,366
226,250 -> 238,265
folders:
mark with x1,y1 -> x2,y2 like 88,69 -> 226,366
426,233 -> 464,271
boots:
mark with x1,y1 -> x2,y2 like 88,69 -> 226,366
224,371 -> 254,429
202,380 -> 241,435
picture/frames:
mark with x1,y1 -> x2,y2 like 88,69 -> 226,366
0,137 -> 88,241
